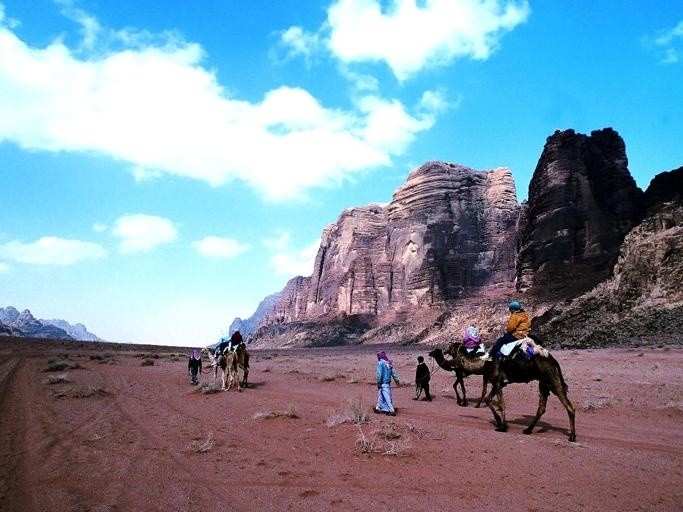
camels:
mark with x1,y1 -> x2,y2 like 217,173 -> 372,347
201,342 -> 250,392
442,340 -> 577,442
429,348 -> 495,409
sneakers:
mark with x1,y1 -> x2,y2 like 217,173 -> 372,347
371,406 -> 379,413
385,411 -> 397,417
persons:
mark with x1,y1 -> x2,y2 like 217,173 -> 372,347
462,323 -> 480,356
372,351 -> 399,416
479,302 -> 529,362
187,348 -> 201,385
411,356 -> 432,401
231,328 -> 243,350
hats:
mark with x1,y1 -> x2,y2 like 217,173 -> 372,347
505,301 -> 521,309
